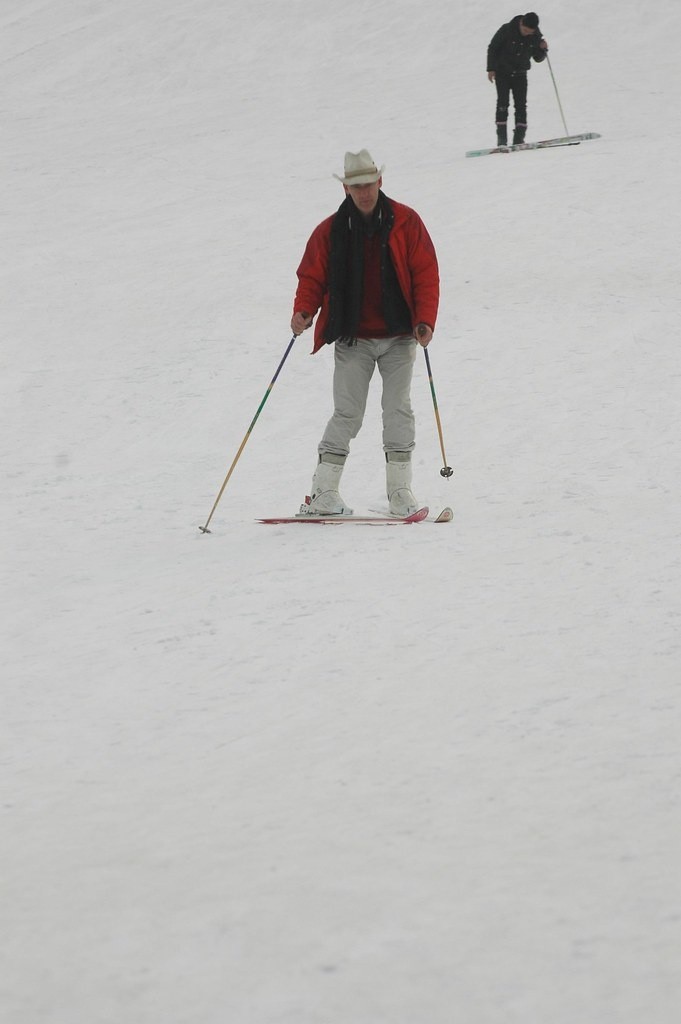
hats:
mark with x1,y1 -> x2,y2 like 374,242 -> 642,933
333,148 -> 386,187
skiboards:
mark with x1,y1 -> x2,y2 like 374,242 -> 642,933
465,132 -> 601,158
254,503 -> 454,526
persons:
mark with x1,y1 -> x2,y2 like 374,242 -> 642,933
290,149 -> 439,520
485,11 -> 548,148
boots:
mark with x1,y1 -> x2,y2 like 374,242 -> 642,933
385,450 -> 419,516
495,121 -> 507,147
512,123 -> 527,145
307,453 -> 354,515
